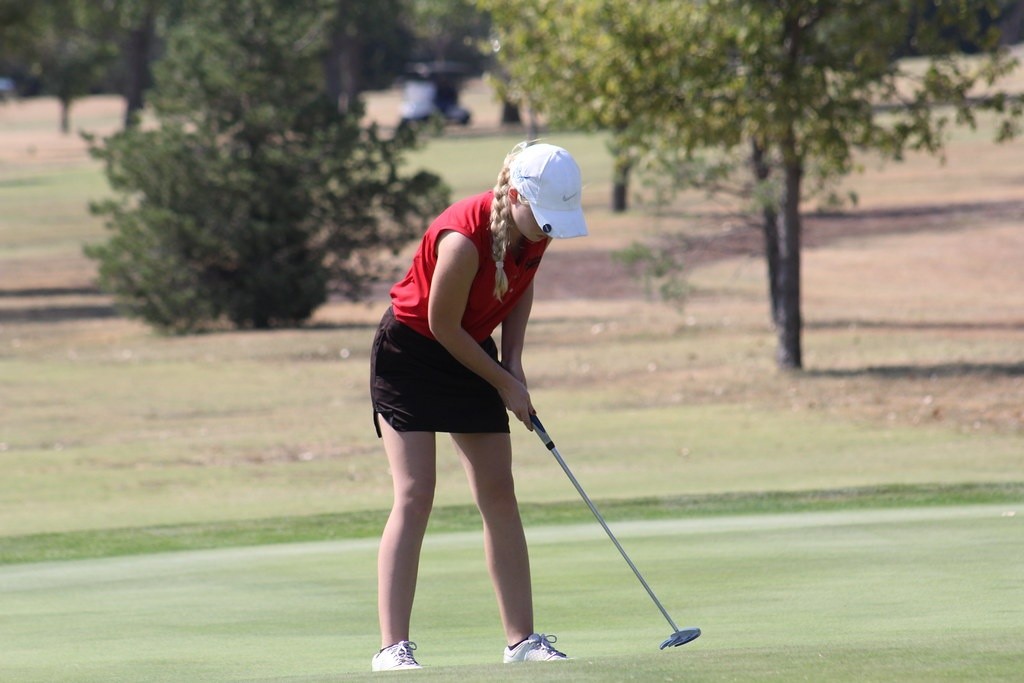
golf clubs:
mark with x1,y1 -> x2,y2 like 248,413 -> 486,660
531,413 -> 702,649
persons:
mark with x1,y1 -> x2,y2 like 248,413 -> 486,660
400,63 -> 458,121
369,143 -> 589,671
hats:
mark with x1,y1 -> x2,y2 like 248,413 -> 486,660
509,141 -> 589,240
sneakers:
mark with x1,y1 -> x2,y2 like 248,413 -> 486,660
504,634 -> 568,665
371,640 -> 422,671
